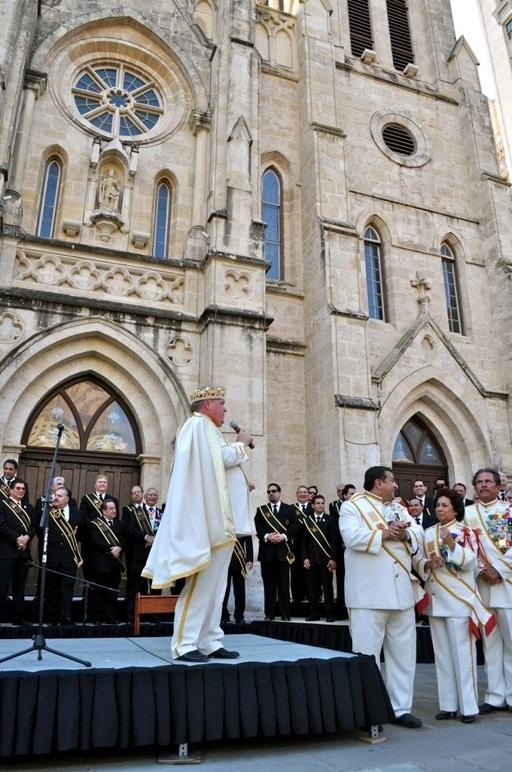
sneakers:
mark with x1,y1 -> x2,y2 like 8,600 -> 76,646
12,612 -> 157,628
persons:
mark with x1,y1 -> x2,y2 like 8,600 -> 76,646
412,490 -> 479,722
221,535 -> 253,623
254,471 -> 512,622
0,459 -> 185,627
459,468 -> 512,714
339,466 -> 425,728
141,384 -> 253,662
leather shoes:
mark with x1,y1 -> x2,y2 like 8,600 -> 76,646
461,716 -> 474,722
436,711 -> 457,720
236,597 -> 339,623
177,650 -> 209,662
208,648 -> 240,658
392,713 -> 421,727
479,703 -> 507,715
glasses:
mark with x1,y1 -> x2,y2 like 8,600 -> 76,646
267,490 -> 280,494
308,491 -> 317,496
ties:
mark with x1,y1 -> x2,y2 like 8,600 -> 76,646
150,507 -> 152,517
273,505 -> 277,517
99,493 -> 114,531
6,479 -> 10,488
316,515 -> 321,526
60,508 -> 64,518
301,504 -> 305,513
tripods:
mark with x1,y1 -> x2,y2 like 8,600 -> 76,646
0,503 -> 93,668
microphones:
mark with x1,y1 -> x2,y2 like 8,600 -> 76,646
229,420 -> 255,450
